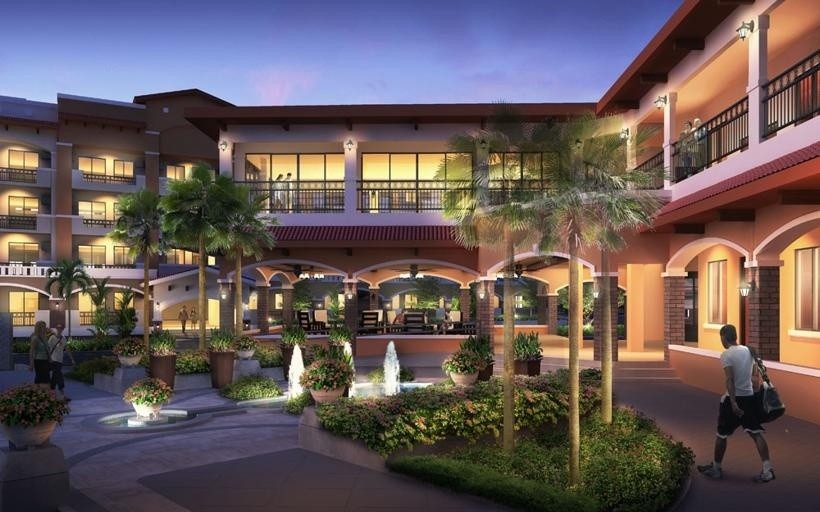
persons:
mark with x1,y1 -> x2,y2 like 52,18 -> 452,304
190,307 -> 197,331
697,325 -> 775,483
178,306 -> 188,333
28,321 -> 48,382
690,118 -> 705,171
678,122 -> 695,177
47,322 -> 75,402
272,175 -> 284,209
284,173 -> 293,213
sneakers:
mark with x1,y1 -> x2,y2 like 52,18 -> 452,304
755,467 -> 775,483
697,465 -> 722,479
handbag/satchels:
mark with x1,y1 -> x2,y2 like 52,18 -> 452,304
760,384 -> 785,422
